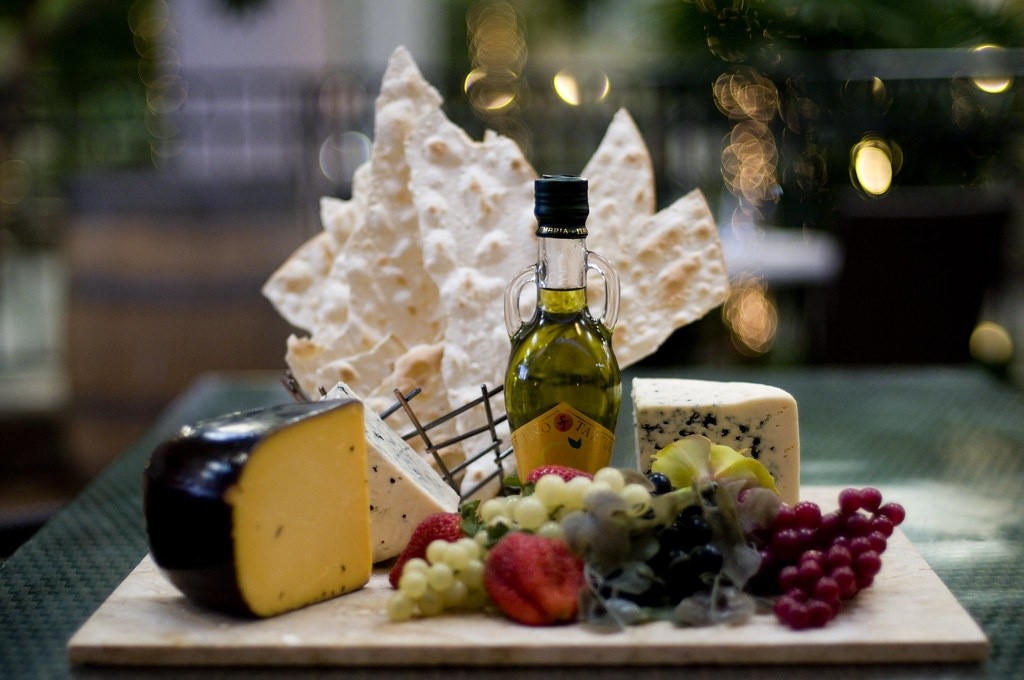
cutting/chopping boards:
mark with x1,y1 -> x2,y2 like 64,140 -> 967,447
61,526 -> 991,666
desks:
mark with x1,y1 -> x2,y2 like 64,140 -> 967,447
0,357 -> 1024,680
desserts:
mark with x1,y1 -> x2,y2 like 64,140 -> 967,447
143,398 -> 374,618
630,379 -> 799,505
317,383 -> 461,563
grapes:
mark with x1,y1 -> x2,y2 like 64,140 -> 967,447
753,487 -> 908,630
388,466 -> 654,623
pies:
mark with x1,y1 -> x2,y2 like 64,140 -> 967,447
260,44 -> 732,498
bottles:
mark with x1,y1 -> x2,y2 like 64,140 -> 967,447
502,173 -> 620,483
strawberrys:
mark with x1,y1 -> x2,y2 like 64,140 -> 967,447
390,511 -> 465,588
530,466 -> 594,485
485,532 -> 582,625
